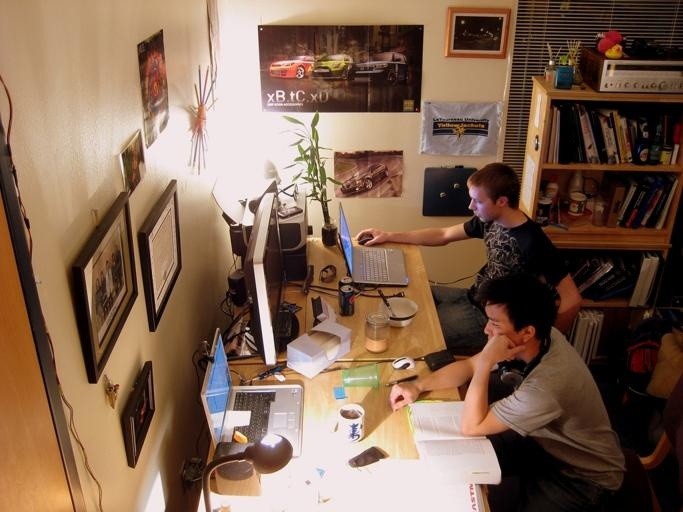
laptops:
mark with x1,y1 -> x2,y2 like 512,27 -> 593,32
338,200 -> 409,287
199,328 -> 304,458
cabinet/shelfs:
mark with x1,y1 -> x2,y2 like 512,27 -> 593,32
517,73 -> 682,363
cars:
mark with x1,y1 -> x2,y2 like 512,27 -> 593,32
347,52 -> 409,87
308,54 -> 353,81
340,163 -> 389,194
268,55 -> 314,79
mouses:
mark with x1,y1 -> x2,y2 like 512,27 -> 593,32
358,234 -> 374,244
392,356 -> 416,370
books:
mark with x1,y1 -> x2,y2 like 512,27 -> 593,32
407,399 -> 502,485
545,105 -> 680,308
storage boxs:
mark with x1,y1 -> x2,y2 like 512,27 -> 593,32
579,44 -> 682,94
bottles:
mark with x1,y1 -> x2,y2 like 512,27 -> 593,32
543,60 -> 584,89
364,310 -> 389,354
633,118 -> 673,166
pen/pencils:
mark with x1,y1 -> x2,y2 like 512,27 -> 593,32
386,375 -> 418,386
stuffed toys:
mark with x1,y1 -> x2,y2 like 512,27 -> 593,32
597,31 -> 623,60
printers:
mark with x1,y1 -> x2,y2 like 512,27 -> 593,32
222,185 -> 313,282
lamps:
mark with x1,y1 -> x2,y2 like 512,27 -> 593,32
200,428 -> 294,511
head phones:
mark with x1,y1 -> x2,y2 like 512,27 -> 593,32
496,327 -> 551,385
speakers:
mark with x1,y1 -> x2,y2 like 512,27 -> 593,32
227,269 -> 246,306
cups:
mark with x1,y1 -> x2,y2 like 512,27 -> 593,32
536,169 -> 609,227
341,363 -> 379,388
337,403 -> 365,444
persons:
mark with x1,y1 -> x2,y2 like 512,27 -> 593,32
389,274 -> 627,512
94,245 -> 124,325
355,163 -> 582,355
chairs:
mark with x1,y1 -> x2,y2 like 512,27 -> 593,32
607,373 -> 681,512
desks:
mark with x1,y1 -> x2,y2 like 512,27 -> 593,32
194,364 -> 494,512
222,232 -> 454,364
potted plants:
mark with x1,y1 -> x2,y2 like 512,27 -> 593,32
281,110 -> 343,247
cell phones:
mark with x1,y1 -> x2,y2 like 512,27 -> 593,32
349,447 -> 384,470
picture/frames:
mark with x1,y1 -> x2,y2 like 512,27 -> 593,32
136,178 -> 182,333
66,189 -> 138,385
442,3 -> 511,60
119,360 -> 156,469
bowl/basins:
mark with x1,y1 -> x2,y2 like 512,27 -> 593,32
378,297 -> 419,327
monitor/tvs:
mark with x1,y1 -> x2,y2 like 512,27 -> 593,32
240,192 -> 299,364
